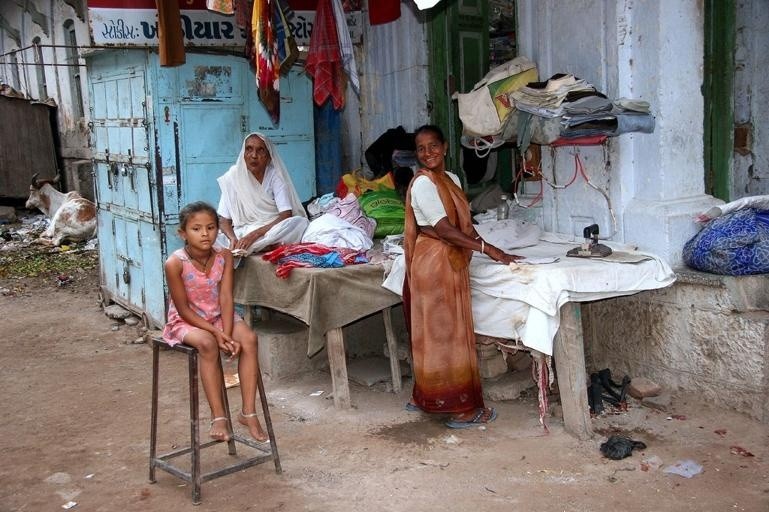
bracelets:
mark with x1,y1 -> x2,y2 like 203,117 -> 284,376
480,240 -> 485,254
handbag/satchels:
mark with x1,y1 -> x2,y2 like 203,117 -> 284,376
335,169 -> 396,198
356,184 -> 408,237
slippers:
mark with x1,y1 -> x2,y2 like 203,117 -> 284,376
406,401 -> 421,412
445,407 -> 497,429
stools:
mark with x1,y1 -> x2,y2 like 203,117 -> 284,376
148,331 -> 280,506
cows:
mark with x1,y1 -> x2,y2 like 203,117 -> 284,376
24,171 -> 98,248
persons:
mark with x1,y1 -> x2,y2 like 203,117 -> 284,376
163,200 -> 269,444
213,132 -> 310,266
403,124 -> 526,429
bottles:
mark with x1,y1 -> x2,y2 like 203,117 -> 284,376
497,194 -> 509,220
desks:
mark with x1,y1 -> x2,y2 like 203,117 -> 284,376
380,229 -> 676,440
234,238 -> 406,412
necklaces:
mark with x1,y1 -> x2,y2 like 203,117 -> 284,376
184,247 -> 211,273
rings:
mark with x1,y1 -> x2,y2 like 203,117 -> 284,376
242,245 -> 244,246
